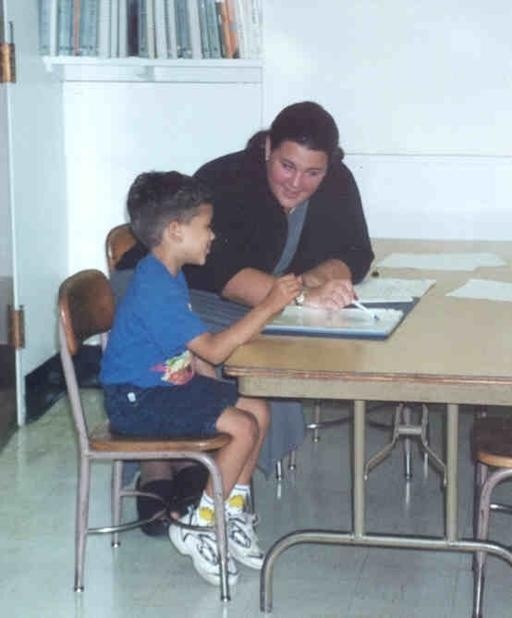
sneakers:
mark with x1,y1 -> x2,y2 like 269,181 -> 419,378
133,472 -> 174,541
222,505 -> 267,573
174,465 -> 213,524
166,503 -> 240,589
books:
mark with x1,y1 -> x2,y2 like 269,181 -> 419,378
260,277 -> 436,339
37,0 -> 260,60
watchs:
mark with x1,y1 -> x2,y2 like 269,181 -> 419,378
295,283 -> 307,305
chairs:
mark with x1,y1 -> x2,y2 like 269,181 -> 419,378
55,267 -> 258,600
470,401 -> 512,618
105,226 -> 283,485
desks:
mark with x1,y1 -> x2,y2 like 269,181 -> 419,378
224,240 -> 512,618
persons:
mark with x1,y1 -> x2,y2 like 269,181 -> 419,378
98,169 -> 264,591
107,100 -> 374,542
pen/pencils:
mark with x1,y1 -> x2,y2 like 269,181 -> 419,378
350,299 -> 380,321
